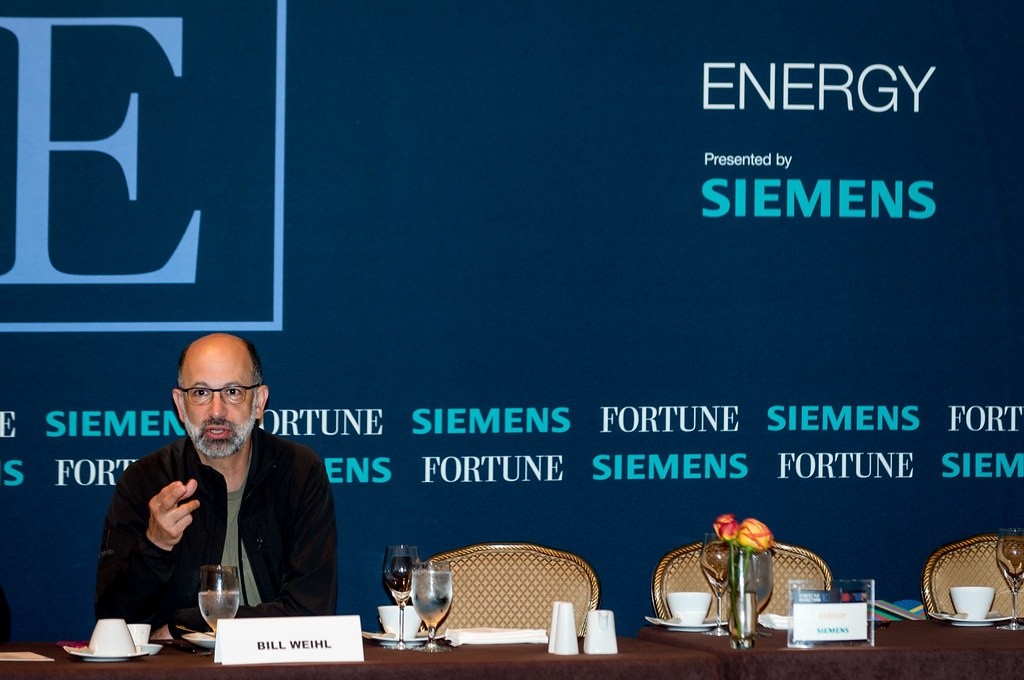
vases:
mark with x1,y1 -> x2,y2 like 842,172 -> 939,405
729,551 -> 756,650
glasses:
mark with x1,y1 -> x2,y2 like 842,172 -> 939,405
179,383 -> 260,406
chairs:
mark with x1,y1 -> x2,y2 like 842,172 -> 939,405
921,534 -> 1024,620
651,541 -> 834,624
418,542 -> 599,638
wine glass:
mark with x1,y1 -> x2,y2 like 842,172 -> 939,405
997,528 -> 1024,630
701,533 -> 728,636
746,550 -> 772,639
383,546 -> 452,653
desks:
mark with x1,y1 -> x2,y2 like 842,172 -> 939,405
0,621 -> 1024,680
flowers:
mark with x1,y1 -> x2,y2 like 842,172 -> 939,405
712,513 -> 775,647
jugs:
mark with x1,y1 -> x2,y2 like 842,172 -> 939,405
583,610 -> 619,654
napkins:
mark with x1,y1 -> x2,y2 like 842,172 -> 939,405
446,627 -> 549,646
759,613 -> 789,631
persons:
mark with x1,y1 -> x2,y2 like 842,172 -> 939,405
95,332 -> 337,640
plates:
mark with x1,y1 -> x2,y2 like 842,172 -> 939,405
645,616 -> 729,632
363,632 -> 446,646
63,644 -> 163,662
182,632 -> 216,648
928,612 -> 1019,626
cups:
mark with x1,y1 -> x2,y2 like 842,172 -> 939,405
950,586 -> 996,620
377,606 -> 423,638
89,619 -> 151,654
667,593 -> 712,625
198,566 -> 239,631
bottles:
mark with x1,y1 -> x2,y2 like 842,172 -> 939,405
548,602 -> 579,656
